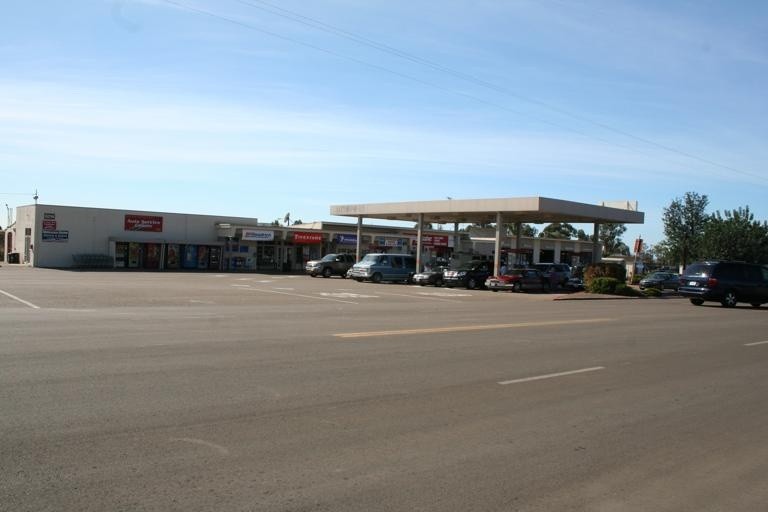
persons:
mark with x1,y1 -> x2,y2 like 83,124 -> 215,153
549,267 -> 556,288
499,263 -> 506,275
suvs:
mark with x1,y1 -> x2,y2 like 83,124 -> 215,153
305,253 -> 355,278
442,260 -> 493,289
679,261 -> 767,307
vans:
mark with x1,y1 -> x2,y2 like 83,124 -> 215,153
351,253 -> 423,284
526,262 -> 571,287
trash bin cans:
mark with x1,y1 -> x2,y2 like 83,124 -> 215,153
7,253 -> 19,264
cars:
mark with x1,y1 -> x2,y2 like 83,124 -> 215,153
639,271 -> 680,290
413,266 -> 445,287
485,269 -> 544,289
